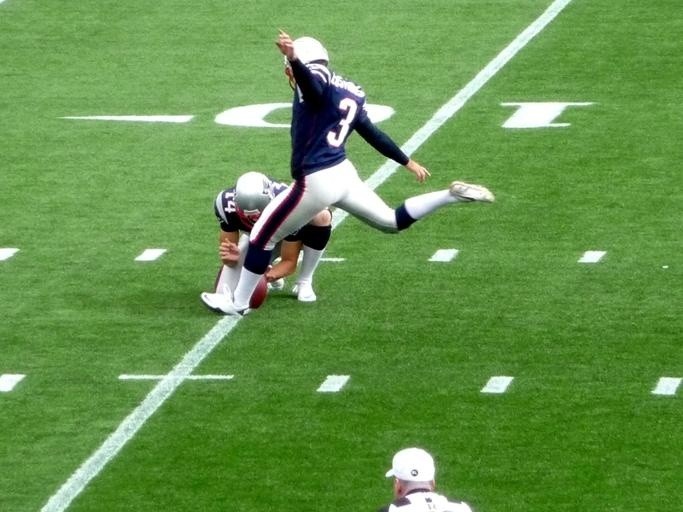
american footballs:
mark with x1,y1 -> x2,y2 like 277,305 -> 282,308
249,274 -> 266,308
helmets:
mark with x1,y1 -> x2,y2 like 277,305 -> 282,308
234,171 -> 275,225
284,36 -> 329,84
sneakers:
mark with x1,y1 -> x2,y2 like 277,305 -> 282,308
449,180 -> 496,205
266,264 -> 316,302
198,291 -> 251,319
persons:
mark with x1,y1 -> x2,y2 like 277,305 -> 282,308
213,171 -> 337,313
200,29 -> 495,315
375,446 -> 473,511
